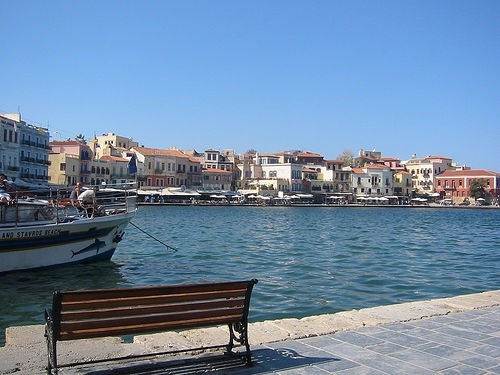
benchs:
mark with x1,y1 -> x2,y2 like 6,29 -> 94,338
43,278 -> 260,375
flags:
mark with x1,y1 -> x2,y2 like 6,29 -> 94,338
127,153 -> 137,175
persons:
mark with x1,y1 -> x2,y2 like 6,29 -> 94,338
145,194 -> 351,204
70,183 -> 86,218
78,186 -> 99,218
0,173 -> 16,207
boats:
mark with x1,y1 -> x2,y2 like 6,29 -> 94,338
0,185 -> 137,275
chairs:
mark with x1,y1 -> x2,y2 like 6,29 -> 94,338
69,198 -> 97,219
